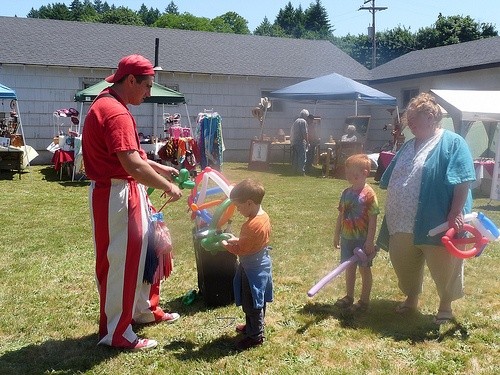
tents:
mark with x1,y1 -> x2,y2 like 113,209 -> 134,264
255,72 -> 401,153
71,75 -> 204,181
0,84 -> 33,179
395,89 -> 500,201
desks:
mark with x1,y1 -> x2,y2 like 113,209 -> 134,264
141,142 -> 165,162
0,147 -> 24,180
471,161 -> 494,194
271,142 -> 291,161
50,148 -> 74,180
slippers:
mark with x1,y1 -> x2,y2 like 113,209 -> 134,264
393,303 -> 421,314
432,315 -> 453,325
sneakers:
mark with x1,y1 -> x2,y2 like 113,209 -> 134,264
125,337 -> 157,351
146,312 -> 181,323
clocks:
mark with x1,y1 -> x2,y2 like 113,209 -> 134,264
248,139 -> 269,162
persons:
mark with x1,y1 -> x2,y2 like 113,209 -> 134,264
138,133 -> 144,140
82,55 -> 183,350
220,178 -> 273,346
318,125 -> 358,175
332,154 -> 379,310
289,108 -> 315,176
374,92 -> 476,326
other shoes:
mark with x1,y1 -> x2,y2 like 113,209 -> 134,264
348,299 -> 369,314
335,296 -> 354,308
235,324 -> 246,333
233,335 -> 264,351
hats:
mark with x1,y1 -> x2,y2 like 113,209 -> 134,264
105,55 -> 154,83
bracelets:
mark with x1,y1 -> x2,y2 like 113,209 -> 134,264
160,183 -> 173,198
